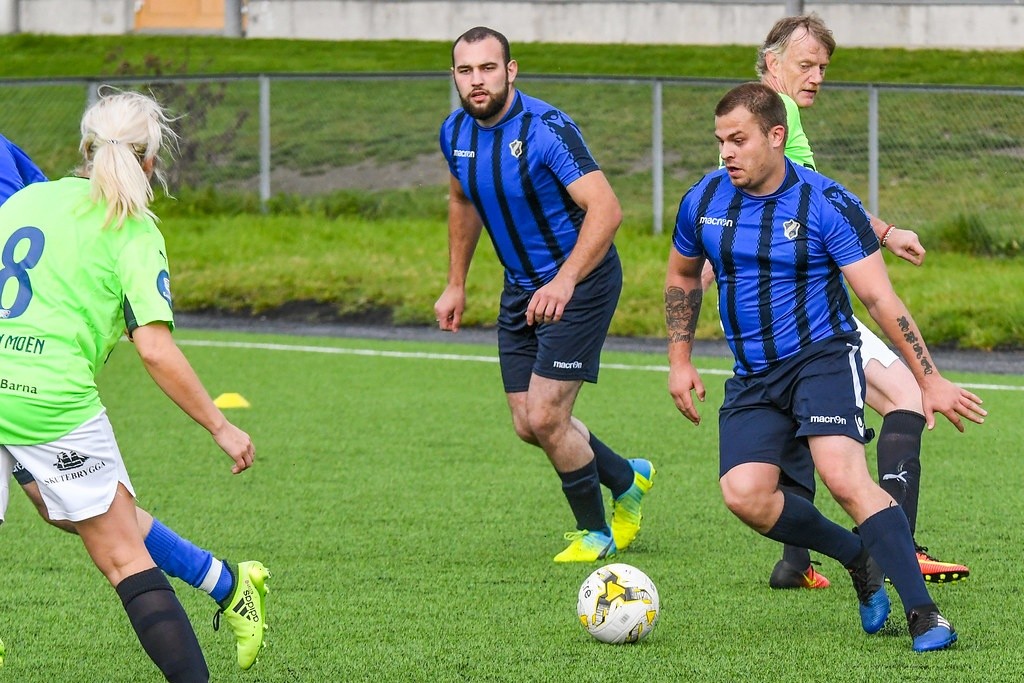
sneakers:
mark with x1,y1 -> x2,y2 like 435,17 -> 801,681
907,601 -> 958,651
553,524 -> 618,562
212,560 -> 271,671
913,538 -> 970,585
768,560 -> 831,588
611,458 -> 657,551
842,526 -> 892,634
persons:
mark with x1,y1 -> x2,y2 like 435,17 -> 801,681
0,84 -> 270,683
700,15 -> 970,589
664,82 -> 988,650
434,28 -> 655,561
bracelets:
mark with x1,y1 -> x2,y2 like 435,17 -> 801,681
878,222 -> 896,246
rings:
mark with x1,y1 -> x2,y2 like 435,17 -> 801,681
435,317 -> 440,323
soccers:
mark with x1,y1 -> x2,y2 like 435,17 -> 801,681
576,563 -> 660,644
